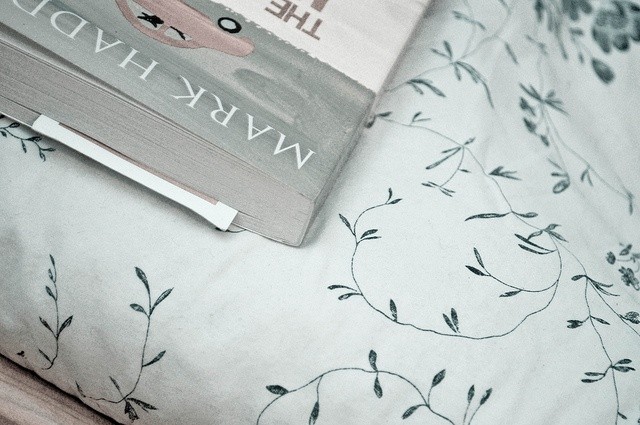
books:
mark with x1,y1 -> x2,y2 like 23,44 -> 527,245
0,1 -> 440,249
